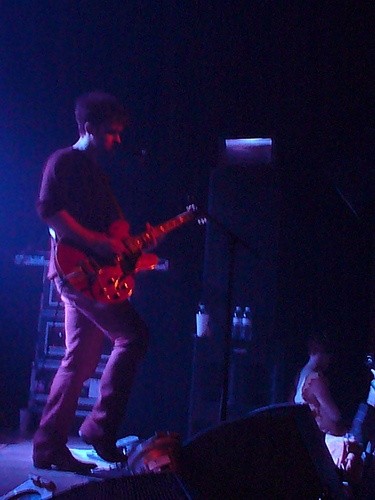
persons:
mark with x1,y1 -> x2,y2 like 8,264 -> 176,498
293,325 -> 375,500
32,91 -> 162,473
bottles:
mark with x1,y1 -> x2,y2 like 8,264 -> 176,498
242,307 -> 253,341
196,304 -> 210,337
232,306 -> 243,339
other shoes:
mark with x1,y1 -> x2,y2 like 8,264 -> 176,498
33,445 -> 97,472
79,430 -> 128,463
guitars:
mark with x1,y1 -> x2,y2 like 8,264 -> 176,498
52,203 -> 209,307
